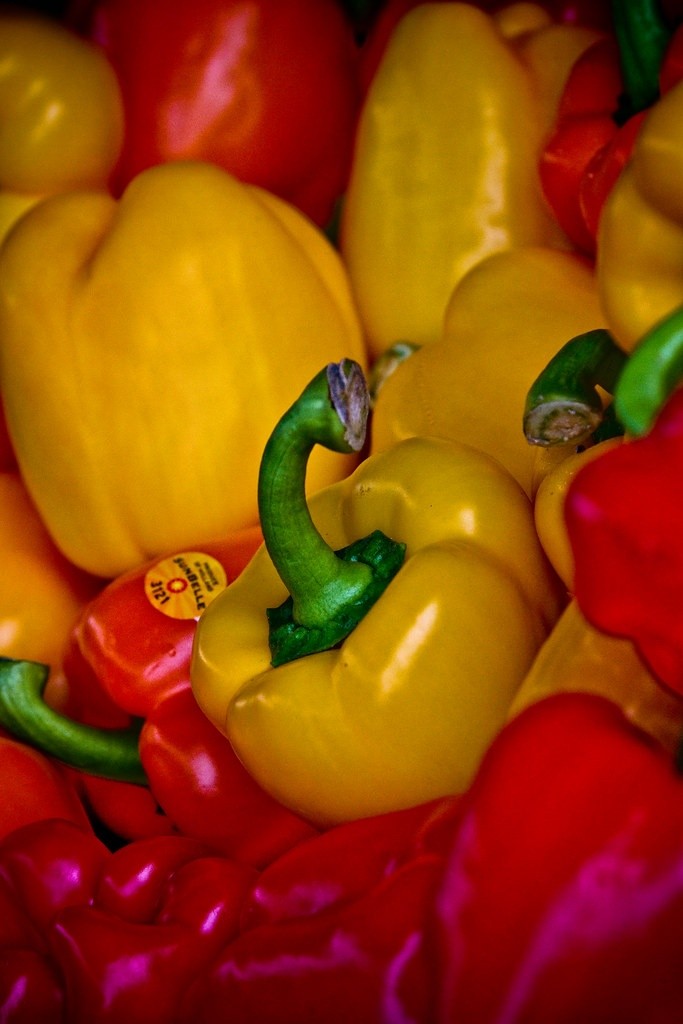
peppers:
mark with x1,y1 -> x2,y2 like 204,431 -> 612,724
0,0 -> 683,1024
